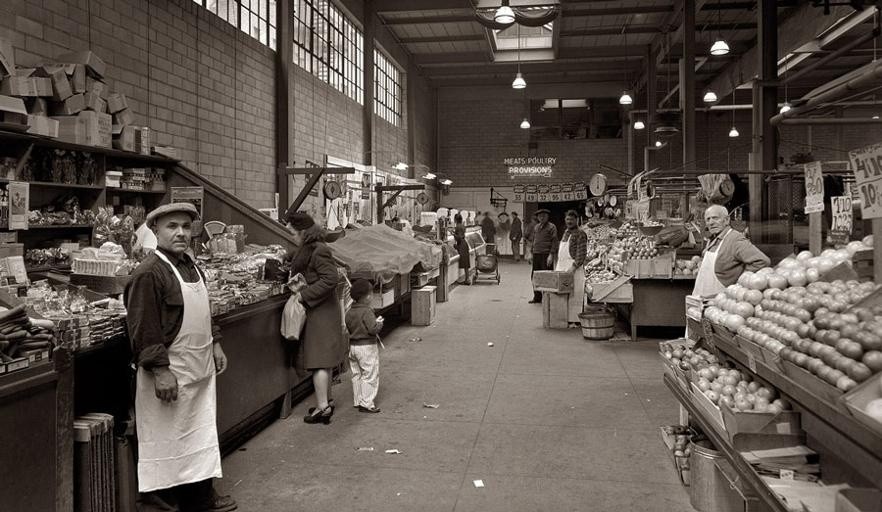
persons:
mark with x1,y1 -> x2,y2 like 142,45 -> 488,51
130,221 -> 158,258
528,208 -> 558,305
344,278 -> 385,412
689,203 -> 772,299
450,213 -> 472,287
481,210 -> 495,253
279,211 -> 346,424
122,202 -> 237,512
554,209 -> 587,329
509,210 -> 521,263
523,214 -> 534,261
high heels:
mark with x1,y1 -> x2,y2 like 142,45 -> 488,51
309,399 -> 335,415
303,406 -> 333,425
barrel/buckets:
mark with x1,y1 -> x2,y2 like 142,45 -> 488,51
690,435 -> 746,511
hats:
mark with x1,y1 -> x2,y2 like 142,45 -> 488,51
534,208 -> 551,216
145,202 -> 199,228
288,212 -> 315,231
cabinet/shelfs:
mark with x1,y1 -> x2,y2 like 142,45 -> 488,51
0,128 -> 170,269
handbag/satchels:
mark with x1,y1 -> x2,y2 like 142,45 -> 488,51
279,293 -> 307,341
264,258 -> 289,283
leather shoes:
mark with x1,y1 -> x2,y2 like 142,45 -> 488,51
210,488 -> 238,511
359,405 -> 380,413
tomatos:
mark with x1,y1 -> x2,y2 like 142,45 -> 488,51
585,223 -> 660,292
705,234 -> 873,332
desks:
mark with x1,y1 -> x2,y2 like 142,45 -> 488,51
214,295 -> 312,445
0,354 -> 77,511
616,280 -> 695,341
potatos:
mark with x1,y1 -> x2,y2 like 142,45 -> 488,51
674,255 -> 702,275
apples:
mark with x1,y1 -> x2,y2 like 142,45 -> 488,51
697,282 -> 882,416
663,424 -> 696,458
663,343 -> 719,369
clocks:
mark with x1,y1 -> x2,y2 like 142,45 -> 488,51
589,173 -> 608,197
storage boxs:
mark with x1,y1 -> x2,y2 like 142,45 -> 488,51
373,288 -> 394,308
659,322 -> 882,484
70,260 -> 133,294
412,286 -> 436,326
587,252 -> 672,304
835,488 -> 882,512
534,271 -> 570,327
0,51 -> 150,154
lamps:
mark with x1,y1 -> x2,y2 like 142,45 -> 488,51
494,0 -> 515,24
520,118 -> 530,129
366,150 -> 453,185
728,89 -> 739,137
633,60 -> 644,129
512,24 -> 526,88
620,32 -> 632,104
710,0 -> 729,55
780,55 -> 791,114
704,31 -> 717,102
